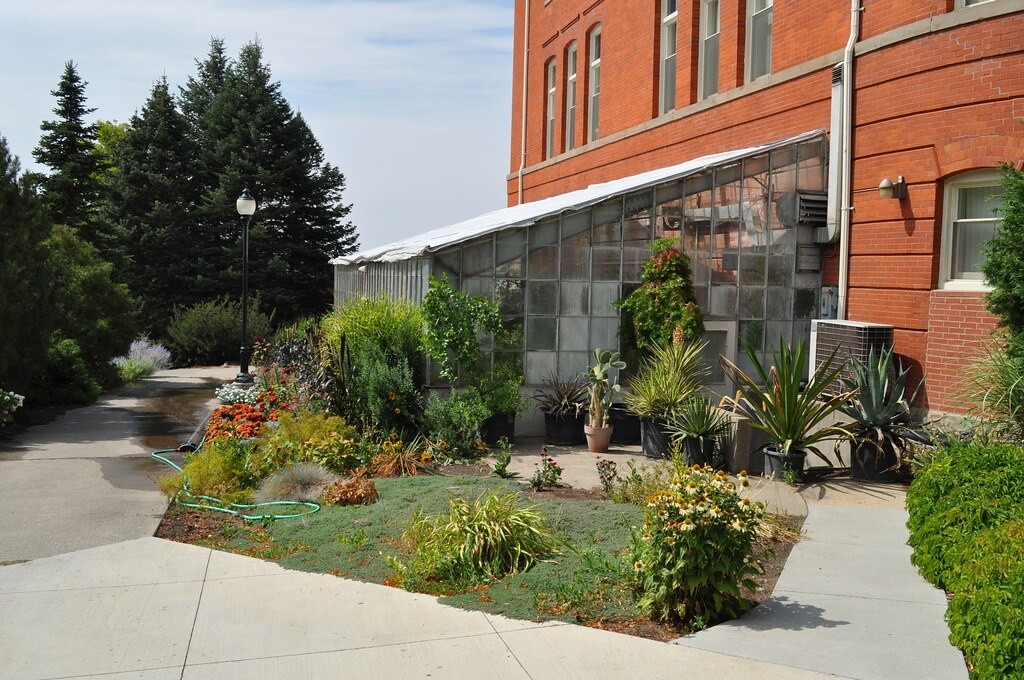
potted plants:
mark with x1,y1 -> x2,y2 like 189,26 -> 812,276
658,394 -> 737,470
832,339 -> 930,482
532,365 -> 592,447
584,347 -> 626,453
477,359 -> 532,449
618,332 -> 713,460
716,332 -> 861,482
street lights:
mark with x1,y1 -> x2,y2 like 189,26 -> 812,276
236,182 -> 257,383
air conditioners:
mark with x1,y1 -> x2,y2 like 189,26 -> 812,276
808,319 -> 895,403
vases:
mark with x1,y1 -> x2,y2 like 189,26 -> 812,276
606,401 -> 643,445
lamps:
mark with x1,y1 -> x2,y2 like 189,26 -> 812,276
875,176 -> 906,201
